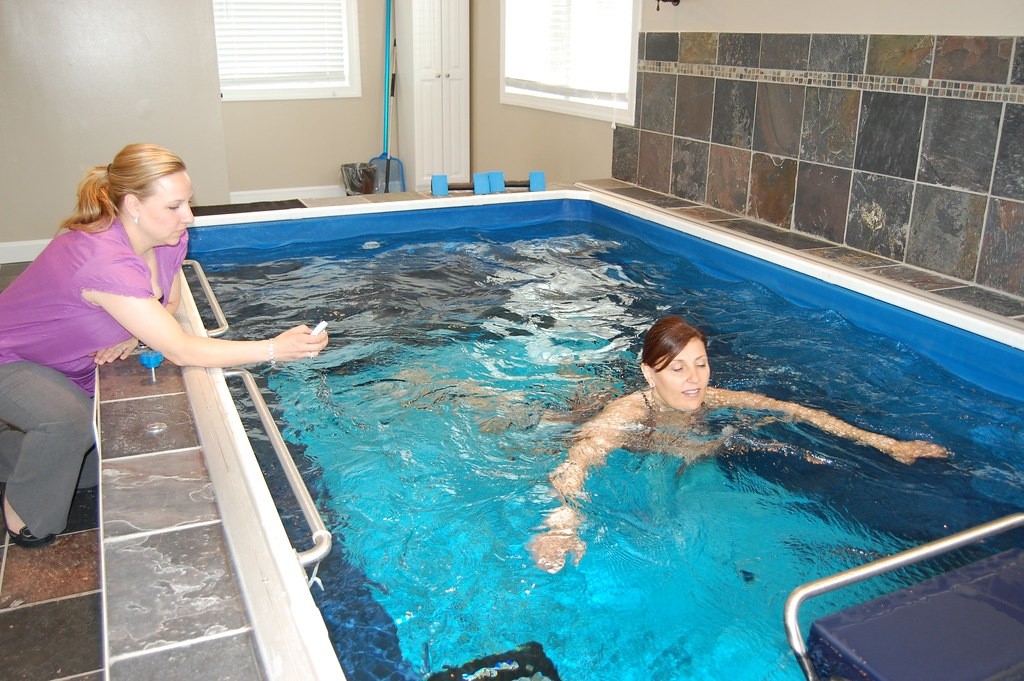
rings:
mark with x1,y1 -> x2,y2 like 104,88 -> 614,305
309,351 -> 314,359
116,348 -> 123,353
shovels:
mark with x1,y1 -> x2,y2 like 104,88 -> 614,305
368,0 -> 406,194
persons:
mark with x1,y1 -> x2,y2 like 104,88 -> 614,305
1,143 -> 330,548
638,317 -> 715,413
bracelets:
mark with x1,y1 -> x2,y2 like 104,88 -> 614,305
269,337 -> 274,362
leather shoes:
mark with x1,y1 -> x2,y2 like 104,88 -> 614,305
0,487 -> 55,548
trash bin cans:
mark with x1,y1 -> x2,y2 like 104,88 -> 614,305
341,163 -> 376,196
